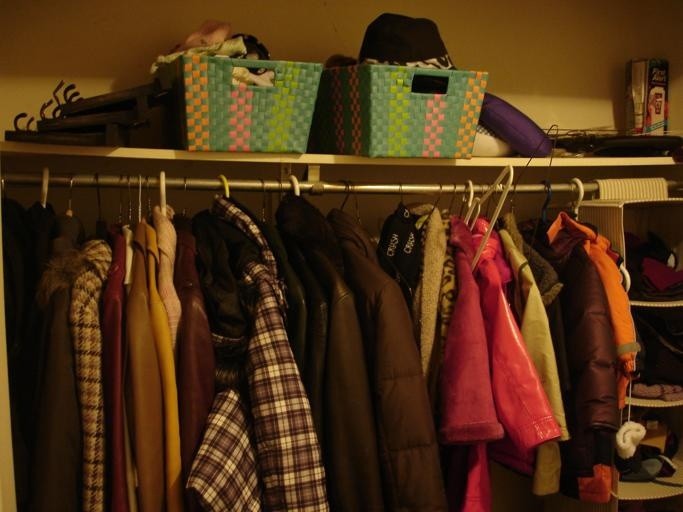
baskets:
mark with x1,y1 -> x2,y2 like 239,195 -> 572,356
309,62 -> 490,159
148,53 -> 328,156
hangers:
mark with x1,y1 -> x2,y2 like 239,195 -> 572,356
13,123 -> 615,313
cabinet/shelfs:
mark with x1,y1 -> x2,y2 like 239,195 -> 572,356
577,197 -> 681,510
